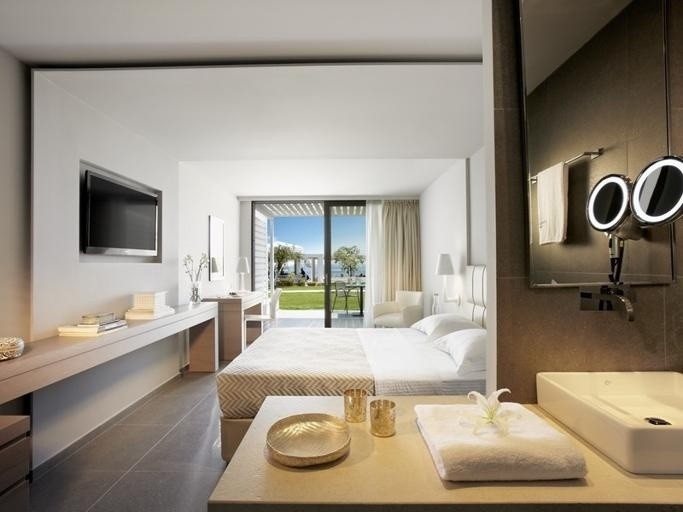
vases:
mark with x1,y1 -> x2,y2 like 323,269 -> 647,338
189,288 -> 201,306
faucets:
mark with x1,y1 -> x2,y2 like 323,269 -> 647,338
583,287 -> 637,321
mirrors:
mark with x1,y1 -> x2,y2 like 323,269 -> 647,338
208,214 -> 224,281
518,0 -> 675,292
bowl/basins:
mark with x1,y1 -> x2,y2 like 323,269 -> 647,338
0,336 -> 24,361
266,414 -> 351,467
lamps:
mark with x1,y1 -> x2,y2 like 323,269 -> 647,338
236,257 -> 249,294
211,256 -> 219,281
435,253 -> 461,306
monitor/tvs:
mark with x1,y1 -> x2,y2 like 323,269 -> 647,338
84,169 -> 159,258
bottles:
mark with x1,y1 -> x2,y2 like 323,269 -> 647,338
431,293 -> 441,316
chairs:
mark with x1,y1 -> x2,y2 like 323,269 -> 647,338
331,280 -> 359,316
372,291 -> 424,328
244,288 -> 281,349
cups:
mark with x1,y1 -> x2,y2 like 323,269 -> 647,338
344,388 -> 396,437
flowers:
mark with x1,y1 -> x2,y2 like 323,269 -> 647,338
183,253 -> 209,302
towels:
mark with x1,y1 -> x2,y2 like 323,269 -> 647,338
413,401 -> 588,481
536,162 -> 569,247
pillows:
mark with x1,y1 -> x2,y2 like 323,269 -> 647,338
408,313 -> 487,374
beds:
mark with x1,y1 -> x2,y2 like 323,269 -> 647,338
215,263 -> 486,466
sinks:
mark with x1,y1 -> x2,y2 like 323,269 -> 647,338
536,369 -> 683,476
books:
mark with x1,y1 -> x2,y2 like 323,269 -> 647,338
56,310 -> 128,338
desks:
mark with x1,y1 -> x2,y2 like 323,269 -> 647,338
344,284 -> 365,317
202,291 -> 265,360
0,301 -> 219,407
